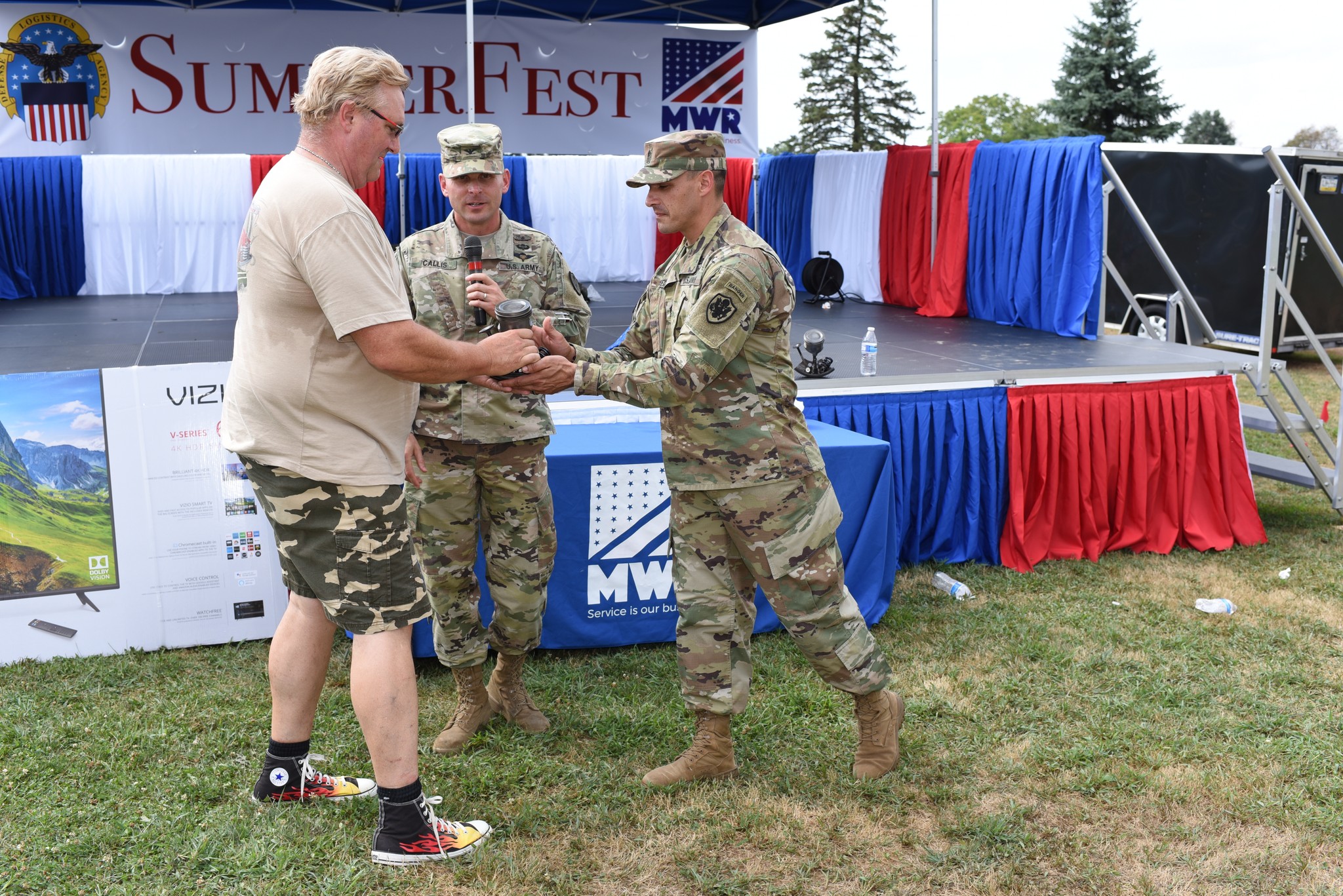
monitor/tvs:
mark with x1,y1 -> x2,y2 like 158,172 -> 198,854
0,368 -> 119,611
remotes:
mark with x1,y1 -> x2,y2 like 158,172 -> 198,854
28,619 -> 77,638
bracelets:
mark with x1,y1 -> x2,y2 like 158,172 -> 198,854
569,344 -> 576,363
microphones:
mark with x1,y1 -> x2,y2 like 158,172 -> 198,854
464,236 -> 487,326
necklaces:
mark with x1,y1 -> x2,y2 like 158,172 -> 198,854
296,144 -> 342,178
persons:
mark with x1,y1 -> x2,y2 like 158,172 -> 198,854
497,131 -> 907,790
392,120 -> 593,755
230,45 -> 542,867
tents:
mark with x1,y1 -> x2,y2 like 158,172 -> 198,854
0,0 -> 942,273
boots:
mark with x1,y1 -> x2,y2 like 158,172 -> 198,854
845,687 -> 906,782
487,651 -> 551,736
368,791 -> 493,867
641,710 -> 739,790
251,748 -> 379,806
432,665 -> 492,758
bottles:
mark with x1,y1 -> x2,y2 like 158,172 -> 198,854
1195,598 -> 1238,616
860,327 -> 878,375
932,571 -> 976,603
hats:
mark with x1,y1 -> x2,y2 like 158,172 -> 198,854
436,123 -> 505,179
625,129 -> 728,189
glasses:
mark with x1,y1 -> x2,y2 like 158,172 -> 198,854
355,101 -> 410,137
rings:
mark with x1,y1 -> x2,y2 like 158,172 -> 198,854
532,390 -> 539,395
